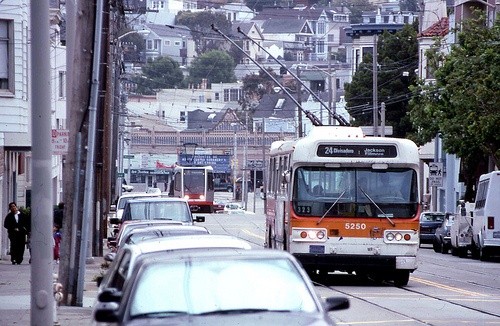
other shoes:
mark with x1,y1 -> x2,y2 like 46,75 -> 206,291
17,263 -> 20,264
12,260 -> 16,265
29,259 -> 32,264
57,258 -> 59,264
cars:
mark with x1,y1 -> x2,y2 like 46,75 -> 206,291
93,193 -> 350,326
121,184 -> 134,192
213,177 -> 234,193
420,199 -> 476,259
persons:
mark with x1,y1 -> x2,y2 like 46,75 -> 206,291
4,201 -> 65,265
350,174 -> 403,197
123,179 -> 127,192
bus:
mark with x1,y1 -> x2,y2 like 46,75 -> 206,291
210,22 -> 423,287
166,142 -> 215,213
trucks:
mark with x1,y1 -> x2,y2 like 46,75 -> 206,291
472,171 -> 500,262
